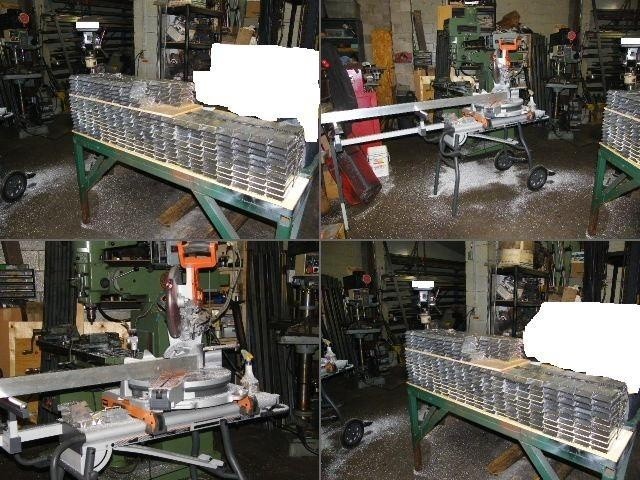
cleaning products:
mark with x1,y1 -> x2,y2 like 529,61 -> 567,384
322,337 -> 337,364
239,349 -> 260,393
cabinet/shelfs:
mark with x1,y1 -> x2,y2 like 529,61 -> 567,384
31,1 -> 263,115
376,251 -> 549,360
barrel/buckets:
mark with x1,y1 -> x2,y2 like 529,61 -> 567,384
393,344 -> 403,364
54,89 -> 69,113
586,104 -> 596,125
595,102 -> 605,125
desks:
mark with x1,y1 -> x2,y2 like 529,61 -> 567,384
64,134 -> 317,241
404,386 -> 640,479
590,147 -> 640,238
